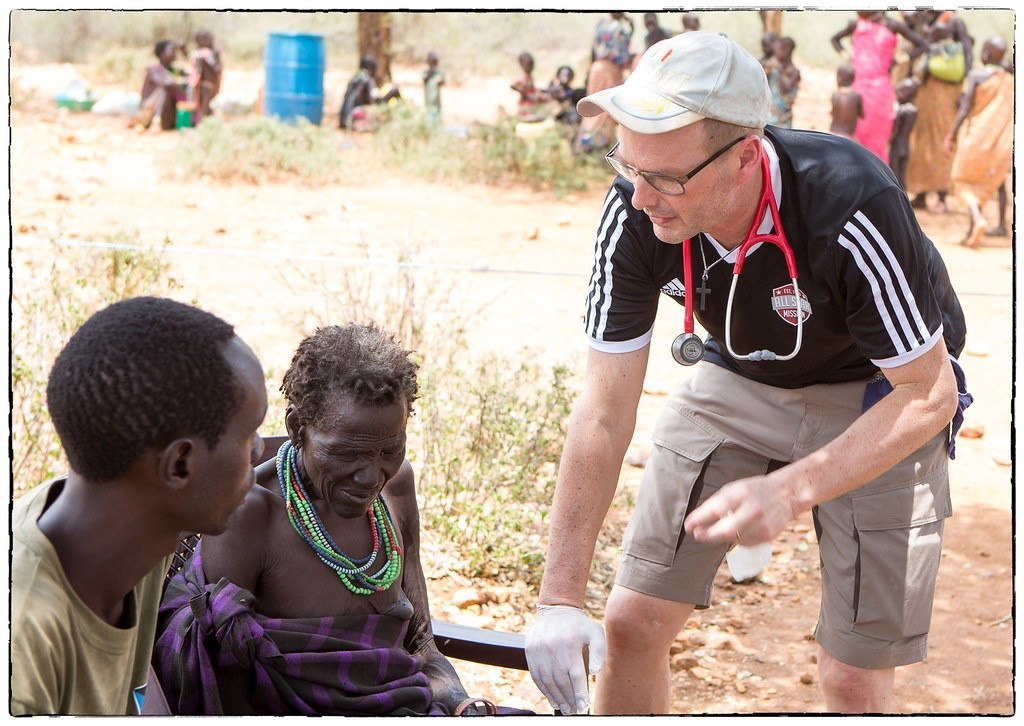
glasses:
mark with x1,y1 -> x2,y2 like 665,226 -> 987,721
604,135 -> 746,197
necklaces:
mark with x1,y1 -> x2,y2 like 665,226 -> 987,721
276,439 -> 401,595
696,206 -> 766,311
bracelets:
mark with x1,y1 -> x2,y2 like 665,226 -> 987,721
454,698 -> 497,716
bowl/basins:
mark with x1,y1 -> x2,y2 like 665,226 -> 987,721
56,99 -> 97,110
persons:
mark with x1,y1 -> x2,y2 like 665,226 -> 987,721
424,53 -> 444,113
192,31 -> 221,123
526,31 -> 972,715
509,8 -> 1016,249
340,55 -> 400,129
11,297 -> 269,718
157,325 -> 499,717
140,39 -> 189,132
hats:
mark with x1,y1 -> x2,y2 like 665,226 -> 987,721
577,32 -> 772,135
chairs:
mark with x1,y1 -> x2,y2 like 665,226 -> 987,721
136,436 -> 592,717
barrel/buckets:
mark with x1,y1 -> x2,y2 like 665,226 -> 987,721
264,33 -> 324,127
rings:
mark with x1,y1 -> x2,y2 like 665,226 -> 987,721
737,532 -> 741,540
